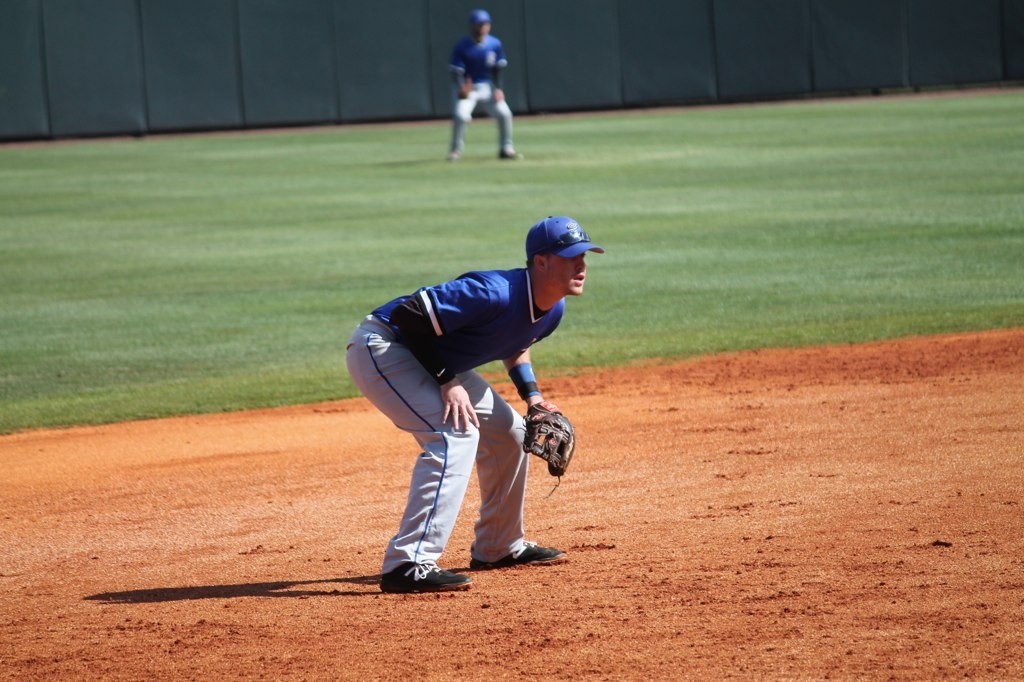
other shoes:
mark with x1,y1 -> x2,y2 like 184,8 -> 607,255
501,149 -> 522,160
447,151 -> 459,160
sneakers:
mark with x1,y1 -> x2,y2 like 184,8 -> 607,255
471,541 -> 565,571
381,562 -> 471,594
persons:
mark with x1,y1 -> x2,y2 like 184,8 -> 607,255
345,215 -> 602,593
445,10 -> 524,161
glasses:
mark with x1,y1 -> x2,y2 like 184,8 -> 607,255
531,231 -> 591,256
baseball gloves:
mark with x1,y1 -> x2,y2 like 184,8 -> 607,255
522,400 -> 575,477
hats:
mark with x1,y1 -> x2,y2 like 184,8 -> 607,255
526,216 -> 604,260
471,10 -> 490,24
